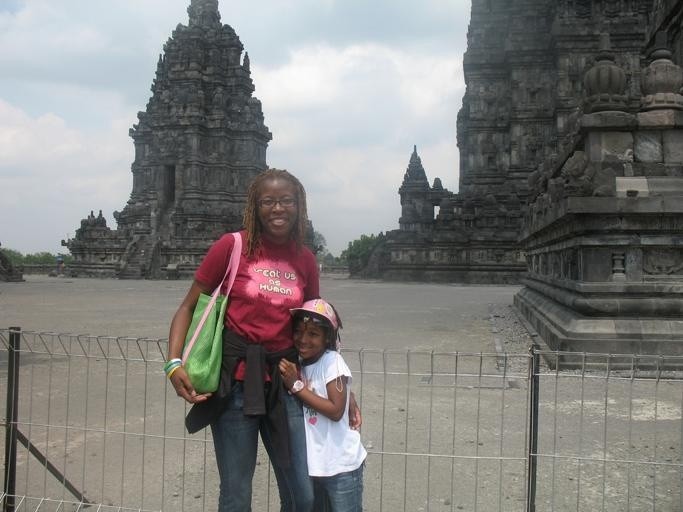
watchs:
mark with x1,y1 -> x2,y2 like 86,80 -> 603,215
288,379 -> 306,396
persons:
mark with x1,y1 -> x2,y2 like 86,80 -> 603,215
163,167 -> 363,511
278,297 -> 367,511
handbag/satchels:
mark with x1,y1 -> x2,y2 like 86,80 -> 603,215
180,232 -> 242,393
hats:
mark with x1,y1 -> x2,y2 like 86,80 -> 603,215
288,299 -> 338,331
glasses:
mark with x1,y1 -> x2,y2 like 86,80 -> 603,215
254,198 -> 297,209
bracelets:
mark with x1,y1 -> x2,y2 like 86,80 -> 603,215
163,358 -> 182,378
